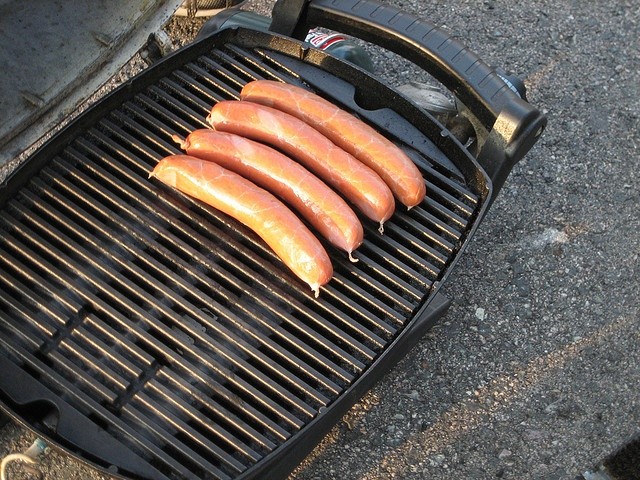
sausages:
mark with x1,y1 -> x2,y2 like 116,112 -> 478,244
171,129 -> 364,263
205,101 -> 396,234
240,80 -> 426,211
148,154 -> 334,298
